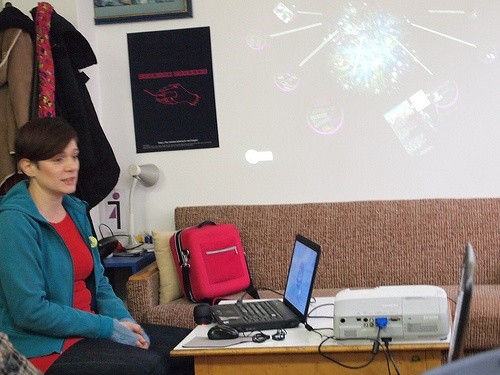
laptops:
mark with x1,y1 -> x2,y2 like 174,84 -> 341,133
212,235 -> 321,331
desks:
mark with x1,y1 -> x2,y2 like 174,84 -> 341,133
170,316 -> 453,375
103,250 -> 154,275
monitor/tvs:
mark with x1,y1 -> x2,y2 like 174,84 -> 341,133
449,242 -> 476,363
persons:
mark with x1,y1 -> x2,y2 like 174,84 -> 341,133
0,116 -> 196,375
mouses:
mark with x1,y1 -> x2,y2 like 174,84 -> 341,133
208,325 -> 239,339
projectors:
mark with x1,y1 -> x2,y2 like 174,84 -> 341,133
334,284 -> 450,342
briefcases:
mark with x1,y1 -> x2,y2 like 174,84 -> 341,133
169,220 -> 253,304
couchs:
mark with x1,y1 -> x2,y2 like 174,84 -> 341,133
127,198 -> 500,360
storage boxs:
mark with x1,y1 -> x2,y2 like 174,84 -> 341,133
170,221 -> 250,304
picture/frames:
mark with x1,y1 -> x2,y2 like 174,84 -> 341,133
93,0 -> 192,24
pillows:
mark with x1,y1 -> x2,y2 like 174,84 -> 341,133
151,230 -> 186,306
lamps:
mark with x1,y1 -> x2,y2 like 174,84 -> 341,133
125,163 -> 159,249
